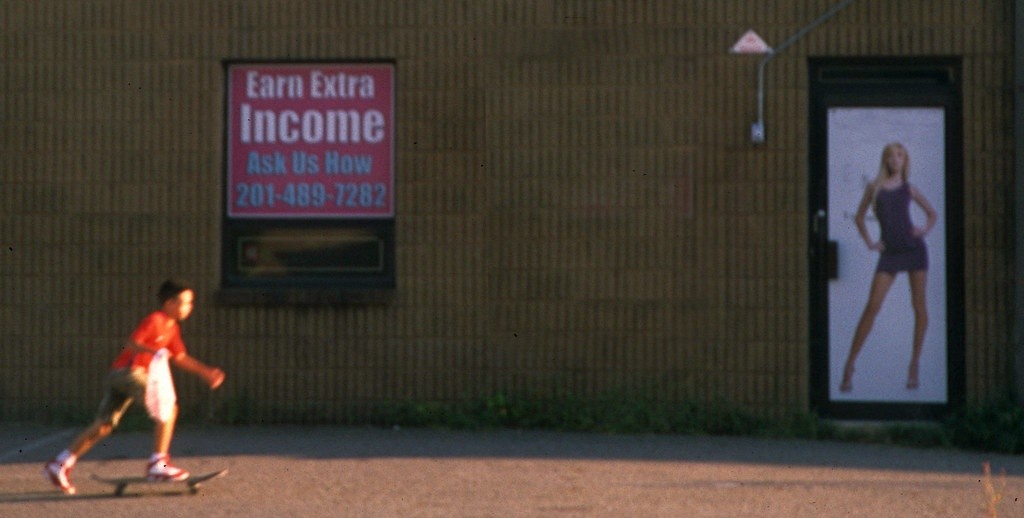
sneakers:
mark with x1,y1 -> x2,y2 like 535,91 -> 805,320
43,461 -> 78,495
146,456 -> 190,482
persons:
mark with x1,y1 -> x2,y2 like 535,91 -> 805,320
44,278 -> 226,495
840,144 -> 938,393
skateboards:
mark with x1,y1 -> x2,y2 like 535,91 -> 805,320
88,470 -> 229,497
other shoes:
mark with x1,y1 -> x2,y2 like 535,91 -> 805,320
839,368 -> 854,391
908,368 -> 919,387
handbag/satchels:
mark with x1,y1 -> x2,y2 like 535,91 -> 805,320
146,348 -> 177,422
